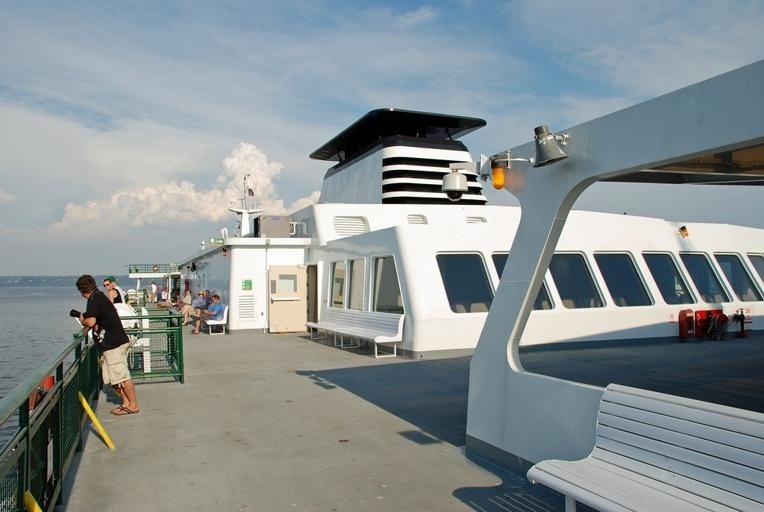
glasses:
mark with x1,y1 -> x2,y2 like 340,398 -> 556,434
103,284 -> 110,287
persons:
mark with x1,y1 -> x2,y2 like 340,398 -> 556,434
74,273 -> 142,417
108,275 -> 126,304
146,281 -> 224,337
103,278 -> 122,304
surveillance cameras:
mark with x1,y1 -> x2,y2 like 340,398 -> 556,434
442,171 -> 469,202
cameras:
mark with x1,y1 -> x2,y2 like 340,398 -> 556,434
69,309 -> 88,319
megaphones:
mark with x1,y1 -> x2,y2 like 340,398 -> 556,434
531,124 -> 569,168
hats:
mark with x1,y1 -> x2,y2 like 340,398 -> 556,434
108,276 -> 116,282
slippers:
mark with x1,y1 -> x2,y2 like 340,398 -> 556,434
110,404 -> 140,415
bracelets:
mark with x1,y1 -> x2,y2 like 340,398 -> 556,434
80,318 -> 84,326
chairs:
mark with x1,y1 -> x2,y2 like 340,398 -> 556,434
203,304 -> 228,335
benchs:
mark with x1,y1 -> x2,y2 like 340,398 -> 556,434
304,308 -> 404,358
524,381 -> 764,511
